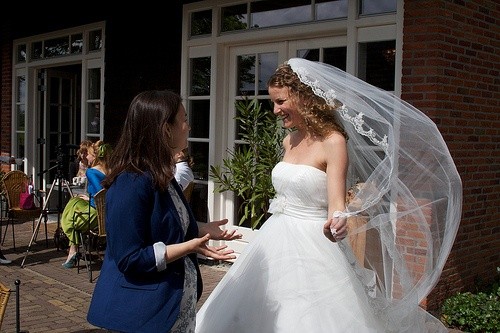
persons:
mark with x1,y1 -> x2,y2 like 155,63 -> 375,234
196,58 -> 463,333
72,139 -> 94,181
171,149 -> 196,195
59,140 -> 115,269
86,90 -> 242,332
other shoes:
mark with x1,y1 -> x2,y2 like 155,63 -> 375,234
0,254 -> 13,265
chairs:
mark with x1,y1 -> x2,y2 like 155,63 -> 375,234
76,188 -> 107,283
183,182 -> 194,204
0,279 -> 21,333
0,170 -> 50,249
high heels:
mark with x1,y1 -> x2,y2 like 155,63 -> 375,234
62,252 -> 80,268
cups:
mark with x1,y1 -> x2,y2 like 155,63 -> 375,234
78,177 -> 86,185
73,177 -> 79,186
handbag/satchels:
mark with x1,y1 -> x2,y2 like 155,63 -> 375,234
19,181 -> 35,210
